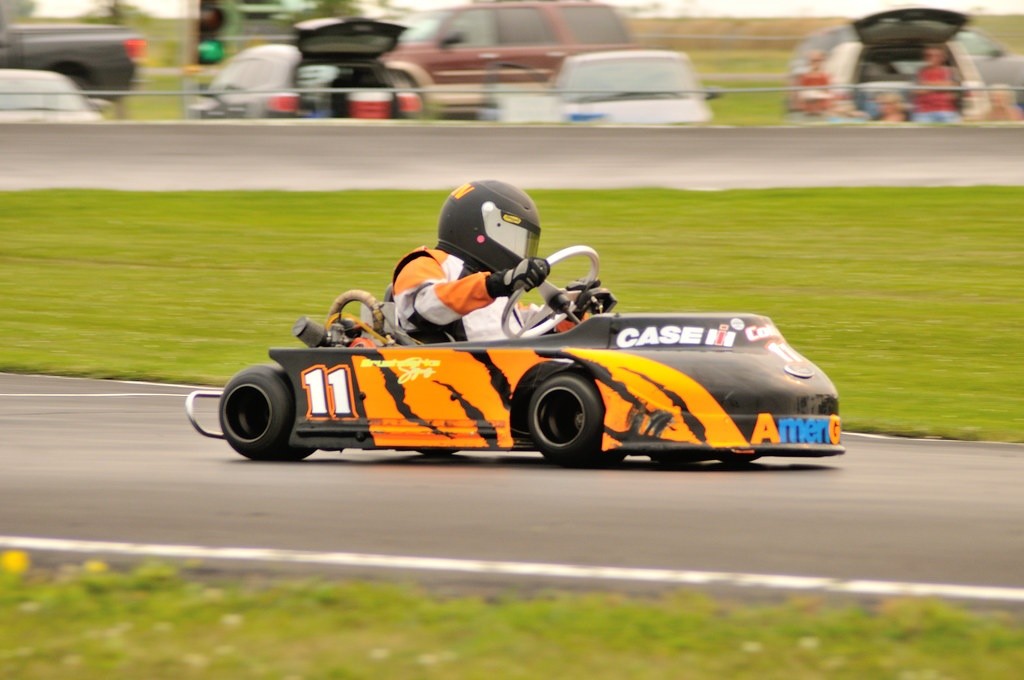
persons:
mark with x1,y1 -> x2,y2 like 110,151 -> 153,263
869,43 -> 1024,123
789,51 -> 836,124
392,180 -> 601,346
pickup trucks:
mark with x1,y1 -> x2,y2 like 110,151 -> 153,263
0,14 -> 142,103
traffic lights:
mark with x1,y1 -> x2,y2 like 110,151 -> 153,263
194,0 -> 226,64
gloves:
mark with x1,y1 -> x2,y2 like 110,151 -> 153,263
484,256 -> 550,300
577,278 -> 618,315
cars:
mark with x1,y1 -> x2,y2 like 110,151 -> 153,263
203,19 -> 429,122
771,7 -> 1020,125
379,2 -> 645,114
473,47 -> 720,125
1,68 -> 108,124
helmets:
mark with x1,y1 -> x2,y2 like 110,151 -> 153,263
435,180 -> 540,274
